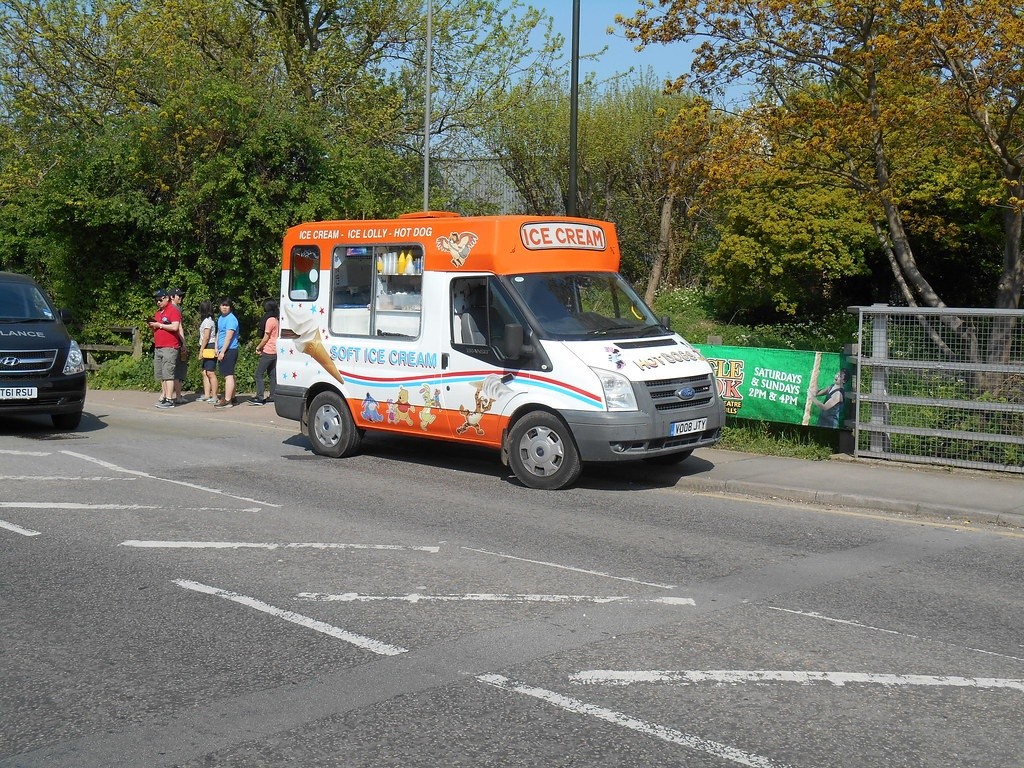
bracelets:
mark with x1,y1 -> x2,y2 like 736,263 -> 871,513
159,324 -> 162,329
255,349 -> 259,351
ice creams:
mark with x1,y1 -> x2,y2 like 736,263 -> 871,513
283,303 -> 344,385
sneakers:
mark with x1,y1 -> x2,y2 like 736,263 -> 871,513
247,395 -> 266,405
158,394 -> 165,402
216,397 -> 240,405
263,396 -> 275,404
206,397 -> 220,403
195,395 -> 211,401
214,397 -> 234,408
155,397 -> 175,409
174,395 -> 189,403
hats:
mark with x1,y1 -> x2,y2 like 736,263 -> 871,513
168,287 -> 185,295
152,290 -> 168,298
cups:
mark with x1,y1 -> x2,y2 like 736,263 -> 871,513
377,249 -> 413,274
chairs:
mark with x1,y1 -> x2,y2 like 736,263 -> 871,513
462,285 -> 505,352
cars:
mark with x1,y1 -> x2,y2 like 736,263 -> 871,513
0,272 -> 86,433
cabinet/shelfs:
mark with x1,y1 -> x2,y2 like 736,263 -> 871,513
375,247 -> 421,338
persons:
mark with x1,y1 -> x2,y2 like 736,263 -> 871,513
247,297 -> 280,404
213,297 -> 240,409
807,367 -> 851,429
196,299 -> 221,403
148,288 -> 189,408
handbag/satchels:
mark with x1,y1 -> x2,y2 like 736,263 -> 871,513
202,348 -> 216,359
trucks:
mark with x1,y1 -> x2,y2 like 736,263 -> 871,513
274,212 -> 726,492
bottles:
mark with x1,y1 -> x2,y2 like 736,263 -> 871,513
414,258 -> 420,274
162,317 -> 171,324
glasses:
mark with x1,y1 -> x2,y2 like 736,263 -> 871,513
154,299 -> 163,303
